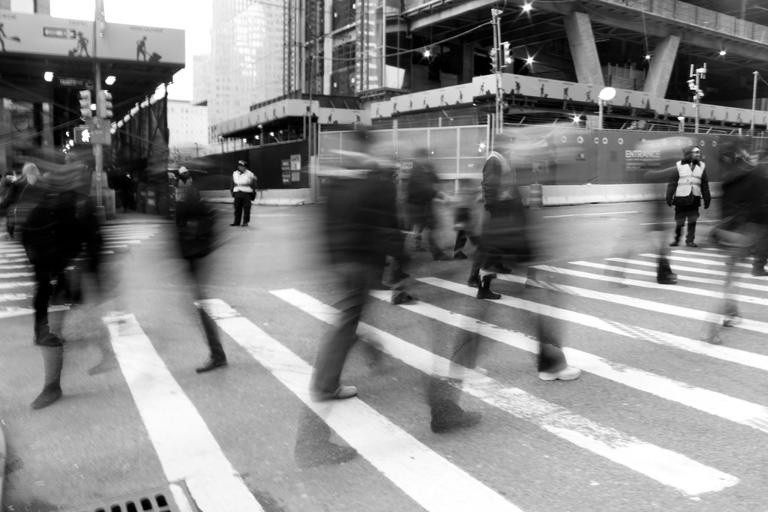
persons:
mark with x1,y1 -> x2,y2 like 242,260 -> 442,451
0,23 -> 11,53
229,157 -> 260,227
75,34 -> 93,59
610,138 -> 767,345
133,36 -> 149,62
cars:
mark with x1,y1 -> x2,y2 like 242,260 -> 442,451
168,172 -> 179,218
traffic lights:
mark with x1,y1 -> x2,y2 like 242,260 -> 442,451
504,42 -> 514,65
74,128 -> 92,144
98,90 -> 113,119
79,90 -> 92,121
488,49 -> 497,72
475,97 -> 497,112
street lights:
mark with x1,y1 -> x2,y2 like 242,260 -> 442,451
270,131 -> 280,143
693,67 -> 707,135
677,113 -> 685,132
257,124 -> 263,145
598,85 -> 615,130
194,142 -> 199,157
219,136 -> 224,153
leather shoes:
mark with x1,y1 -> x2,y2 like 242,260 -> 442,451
194,353 -> 228,374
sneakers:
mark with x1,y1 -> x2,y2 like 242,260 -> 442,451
310,383 -> 359,400
475,287 -> 503,301
658,267 -> 677,286
290,441 -> 357,471
428,409 -> 484,434
536,364 -> 584,383
27,385 -> 64,408
